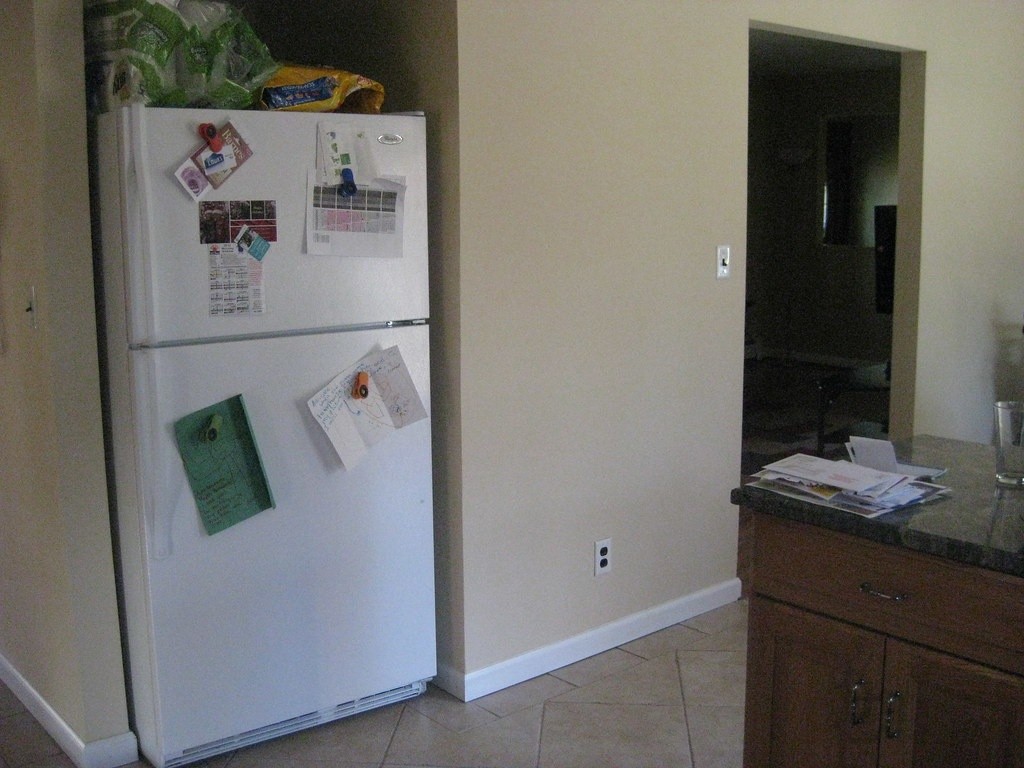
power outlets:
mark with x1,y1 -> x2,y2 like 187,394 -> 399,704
594,536 -> 611,577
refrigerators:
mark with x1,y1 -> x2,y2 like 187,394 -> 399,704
90,101 -> 440,768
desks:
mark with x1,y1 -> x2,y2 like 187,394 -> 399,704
743,344 -> 892,456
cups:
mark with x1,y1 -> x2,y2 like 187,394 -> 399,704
992,400 -> 1024,485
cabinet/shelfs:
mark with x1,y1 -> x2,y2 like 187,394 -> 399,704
735,504 -> 1024,768
